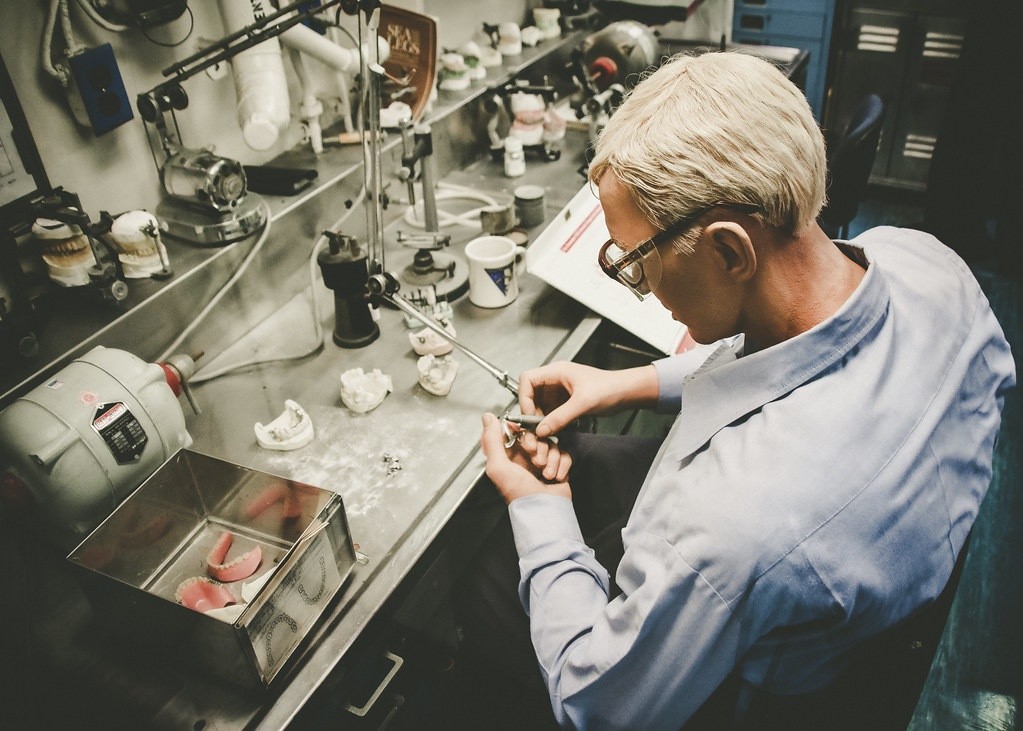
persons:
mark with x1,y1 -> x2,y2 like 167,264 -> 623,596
483,54 -> 1017,731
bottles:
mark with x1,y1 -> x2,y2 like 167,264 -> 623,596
504,137 -> 525,178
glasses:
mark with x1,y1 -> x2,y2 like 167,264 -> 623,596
598,200 -> 762,302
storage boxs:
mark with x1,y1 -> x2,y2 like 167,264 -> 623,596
68,446 -> 357,696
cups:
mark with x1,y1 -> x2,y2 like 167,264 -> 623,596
464,236 -> 528,310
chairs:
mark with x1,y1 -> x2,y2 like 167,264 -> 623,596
815,95 -> 884,238
904,380 -> 1023,731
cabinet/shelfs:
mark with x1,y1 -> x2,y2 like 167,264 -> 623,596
732,0 -> 973,193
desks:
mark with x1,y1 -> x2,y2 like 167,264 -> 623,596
0,47 -> 812,731
